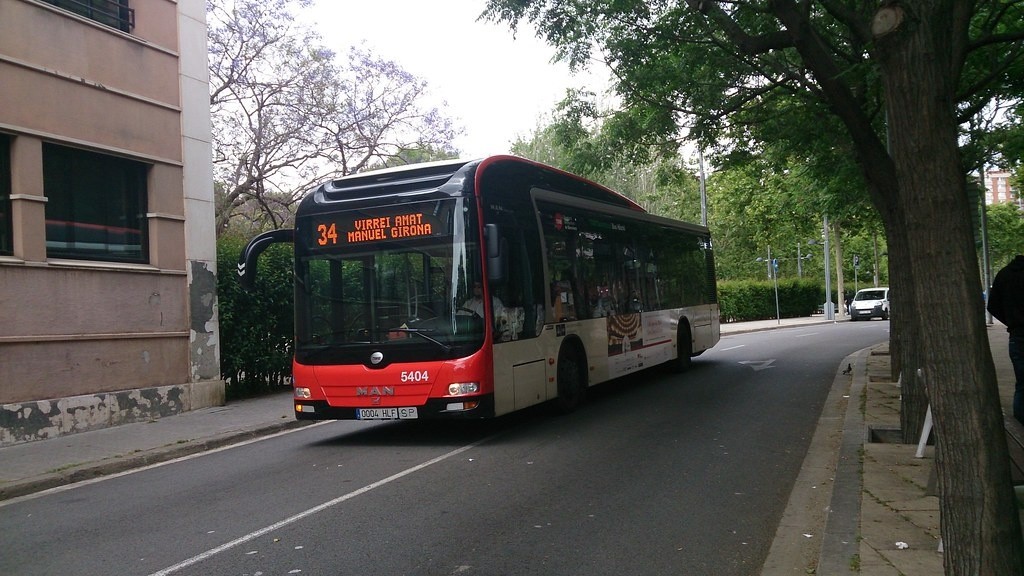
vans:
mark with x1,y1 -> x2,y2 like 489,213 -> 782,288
849,287 -> 890,321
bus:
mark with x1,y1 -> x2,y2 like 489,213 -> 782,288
234,153 -> 723,426
0,210 -> 148,265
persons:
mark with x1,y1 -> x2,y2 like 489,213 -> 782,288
986,255 -> 1024,424
456,279 -> 505,328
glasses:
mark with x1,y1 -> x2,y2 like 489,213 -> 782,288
472,284 -> 483,288
600,288 -> 609,294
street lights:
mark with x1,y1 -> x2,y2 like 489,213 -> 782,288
755,253 -> 813,325
807,238 -> 831,320
